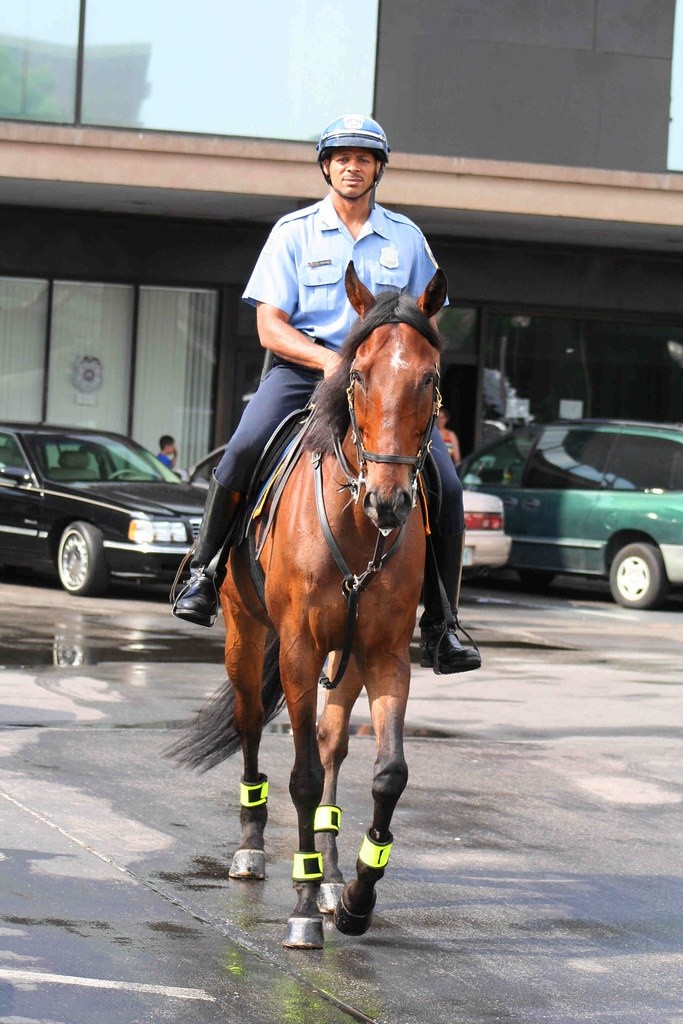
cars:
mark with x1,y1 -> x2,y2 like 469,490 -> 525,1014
456,421 -> 683,609
186,438 -> 511,578
0,421 -> 212,594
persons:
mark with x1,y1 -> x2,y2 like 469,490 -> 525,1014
172,114 -> 486,678
435,409 -> 460,465
151,435 -> 178,482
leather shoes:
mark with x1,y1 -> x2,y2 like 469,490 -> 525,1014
175,575 -> 218,627
419,622 -> 481,675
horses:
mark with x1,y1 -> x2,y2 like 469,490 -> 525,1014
159,260 -> 450,952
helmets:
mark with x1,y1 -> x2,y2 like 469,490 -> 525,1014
316,113 -> 391,166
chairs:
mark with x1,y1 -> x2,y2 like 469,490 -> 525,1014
47,451 -> 97,482
